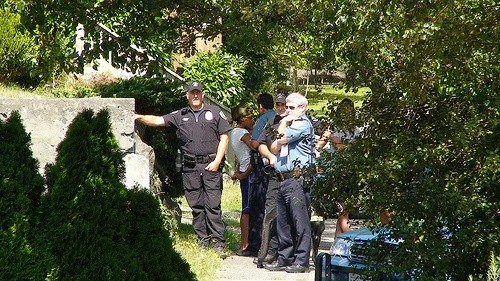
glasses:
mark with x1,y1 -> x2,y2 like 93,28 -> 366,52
284,105 -> 295,110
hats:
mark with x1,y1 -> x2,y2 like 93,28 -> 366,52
187,81 -> 202,91
233,106 -> 250,121
276,91 -> 288,103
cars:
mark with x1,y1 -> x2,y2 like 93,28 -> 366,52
322,224 -> 455,281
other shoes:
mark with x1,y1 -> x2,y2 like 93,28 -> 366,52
263,260 -> 288,271
237,244 -> 259,257
198,240 -> 209,251
253,255 -> 278,265
211,241 -> 226,253
286,263 -> 310,274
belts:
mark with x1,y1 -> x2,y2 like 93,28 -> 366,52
278,172 -> 303,179
182,152 -> 216,164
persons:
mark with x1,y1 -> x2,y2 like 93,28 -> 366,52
230,93 -> 292,268
134,83 -> 233,257
264,92 -> 320,273
305,97 -> 392,265
